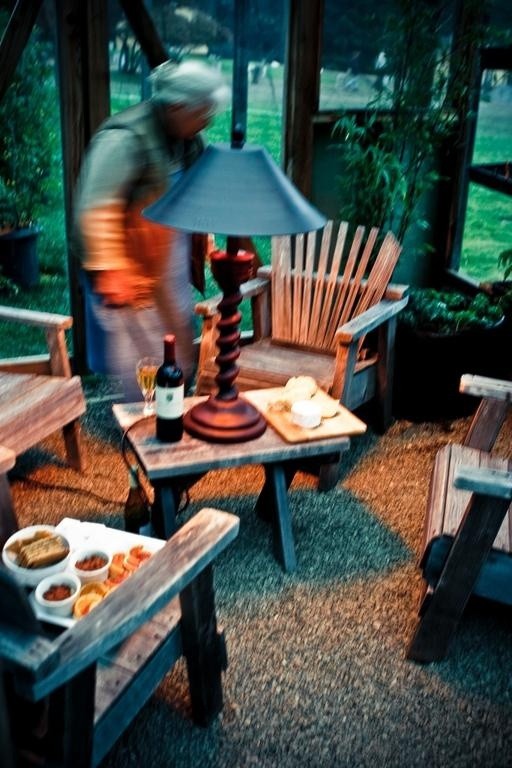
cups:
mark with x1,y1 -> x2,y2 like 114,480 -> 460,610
289,401 -> 321,428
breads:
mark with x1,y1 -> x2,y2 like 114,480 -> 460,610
269,373 -> 341,431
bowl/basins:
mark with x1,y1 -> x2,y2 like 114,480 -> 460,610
34,572 -> 81,616
0,524 -> 73,586
71,546 -> 113,586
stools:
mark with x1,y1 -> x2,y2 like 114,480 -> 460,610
405,373 -> 511,666
0,302 -> 87,534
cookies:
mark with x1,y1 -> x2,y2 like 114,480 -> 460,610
8,531 -> 68,566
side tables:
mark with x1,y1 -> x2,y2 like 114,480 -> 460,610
110,377 -> 369,571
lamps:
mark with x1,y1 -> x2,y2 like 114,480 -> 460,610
137,147 -> 328,440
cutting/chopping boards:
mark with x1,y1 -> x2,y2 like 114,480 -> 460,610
244,385 -> 367,444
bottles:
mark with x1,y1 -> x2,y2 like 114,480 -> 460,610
155,335 -> 184,444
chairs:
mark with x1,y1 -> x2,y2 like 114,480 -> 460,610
193,221 -> 409,496
2,503 -> 239,765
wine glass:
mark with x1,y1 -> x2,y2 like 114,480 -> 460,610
135,358 -> 161,412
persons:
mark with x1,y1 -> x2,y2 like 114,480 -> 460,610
71,58 -> 232,400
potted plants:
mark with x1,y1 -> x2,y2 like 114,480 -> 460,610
397,285 -> 507,422
